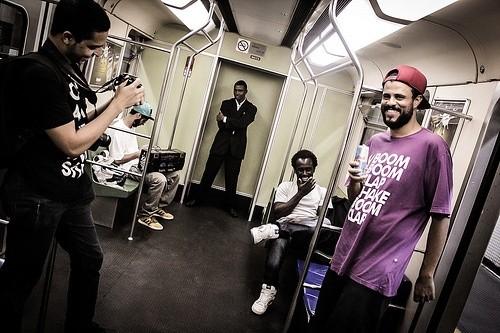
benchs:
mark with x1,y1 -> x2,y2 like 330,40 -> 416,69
297,258 -> 412,333
85,146 -> 140,230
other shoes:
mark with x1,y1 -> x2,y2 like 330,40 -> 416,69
186,199 -> 197,207
229,208 -> 239,218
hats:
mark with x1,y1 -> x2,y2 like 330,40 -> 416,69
382,64 -> 431,110
133,101 -> 155,122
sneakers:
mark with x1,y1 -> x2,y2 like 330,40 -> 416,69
251,284 -> 278,315
137,217 -> 163,231
153,208 -> 174,219
250,223 -> 281,245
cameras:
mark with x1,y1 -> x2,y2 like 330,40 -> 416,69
89,133 -> 111,152
113,73 -> 142,93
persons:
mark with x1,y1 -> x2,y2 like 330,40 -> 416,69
0,0 -> 143,333
305,65 -> 454,333
185,79 -> 258,217
250,150 -> 333,315
99,102 -> 180,231
